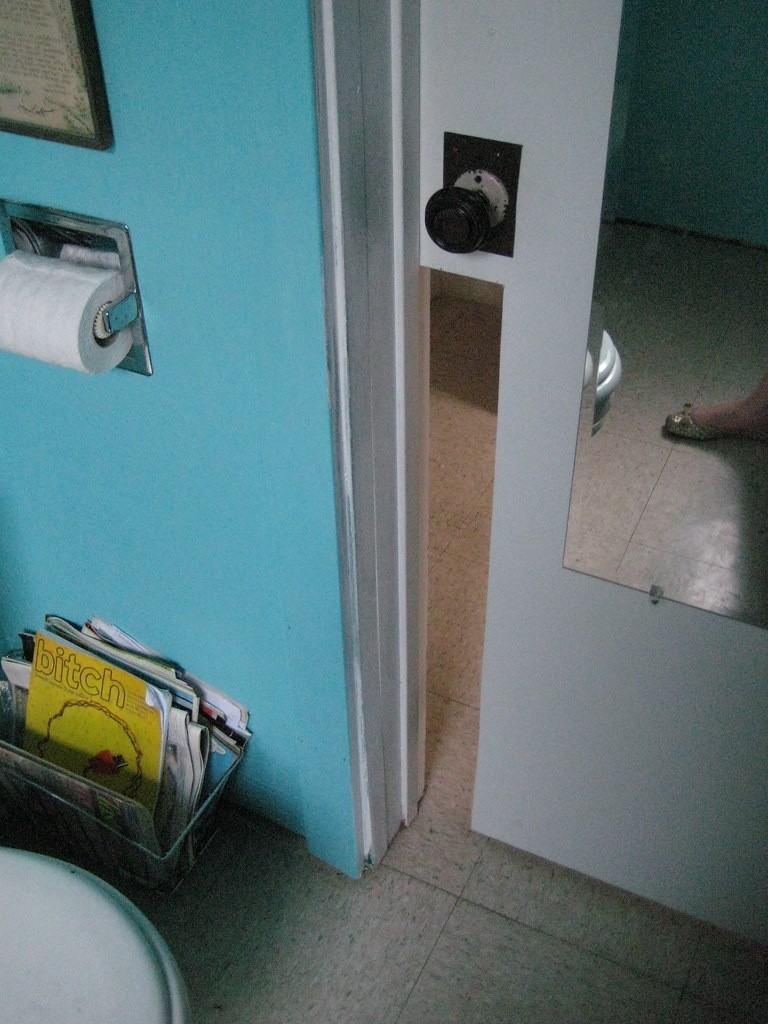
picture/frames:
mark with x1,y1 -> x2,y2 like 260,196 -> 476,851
0,0 -> 114,151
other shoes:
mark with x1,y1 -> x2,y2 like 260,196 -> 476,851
662,396 -> 768,442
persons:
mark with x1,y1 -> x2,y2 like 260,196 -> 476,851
665,372 -> 768,440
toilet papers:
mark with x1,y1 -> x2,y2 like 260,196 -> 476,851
2,250 -> 135,376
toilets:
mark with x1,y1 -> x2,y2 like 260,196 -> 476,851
2,846 -> 196,1024
593,330 -> 624,440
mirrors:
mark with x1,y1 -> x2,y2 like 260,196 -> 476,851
564,0 -> 768,630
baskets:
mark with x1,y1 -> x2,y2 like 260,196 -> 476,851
0,644 -> 244,894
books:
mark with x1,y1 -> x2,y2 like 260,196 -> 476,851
0,615 -> 251,889
